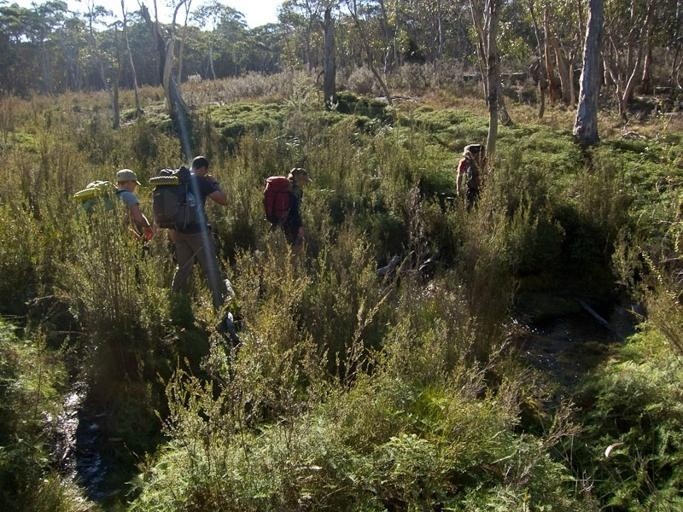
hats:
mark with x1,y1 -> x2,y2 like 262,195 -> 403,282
116,169 -> 143,186
291,167 -> 314,183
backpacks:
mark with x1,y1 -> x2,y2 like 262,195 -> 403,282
149,166 -> 200,233
75,180 -> 129,243
463,143 -> 488,193
262,175 -> 295,227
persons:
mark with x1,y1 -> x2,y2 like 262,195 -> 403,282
455,146 -> 486,212
116,168 -> 146,229
281,167 -> 313,263
171,155 -> 226,294
